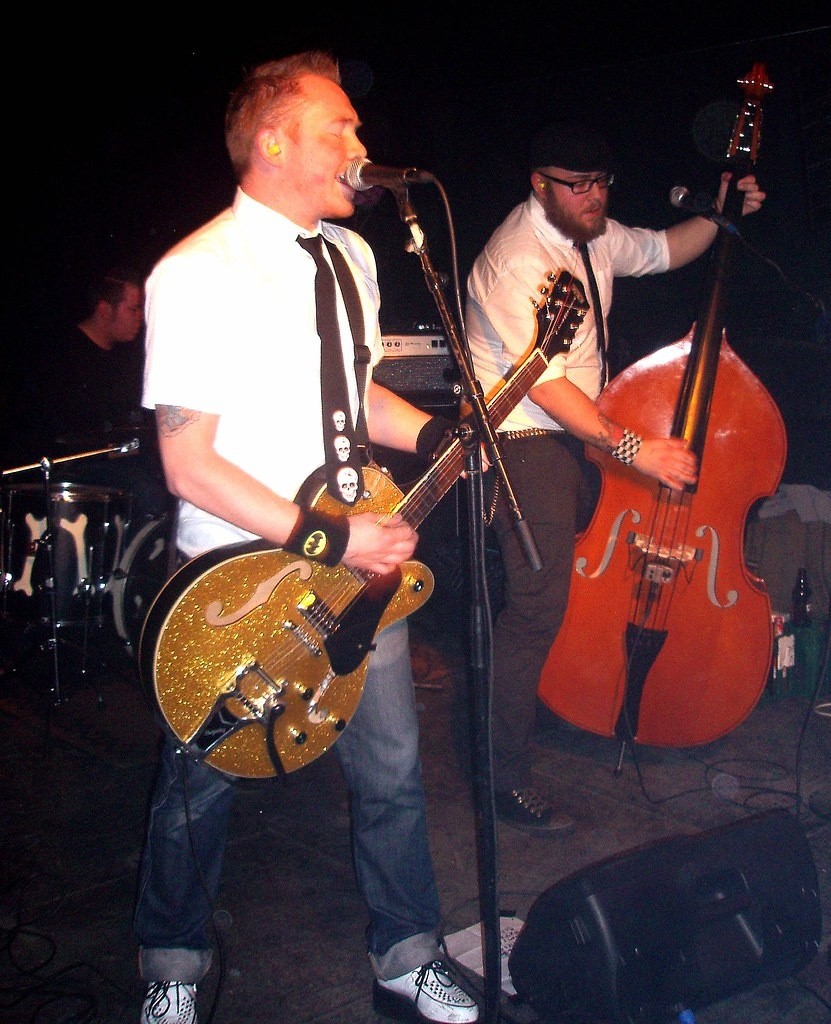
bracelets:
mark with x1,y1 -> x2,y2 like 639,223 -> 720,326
415,417 -> 458,463
282,505 -> 351,569
611,428 -> 643,466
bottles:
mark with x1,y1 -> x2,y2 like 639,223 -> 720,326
770,616 -> 787,679
790,568 -> 813,627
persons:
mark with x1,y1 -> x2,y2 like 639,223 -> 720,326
1,263 -> 174,546
463,136 -> 766,830
134,51 -> 494,1024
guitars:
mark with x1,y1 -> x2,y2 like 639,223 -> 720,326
138,266 -> 591,779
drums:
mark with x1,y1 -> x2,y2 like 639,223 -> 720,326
0,482 -> 134,629
112,510 -> 174,663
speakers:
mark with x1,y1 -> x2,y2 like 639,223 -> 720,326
508,803 -> 822,1024
364,392 -> 512,651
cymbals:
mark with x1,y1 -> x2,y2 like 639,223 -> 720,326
82,423 -> 155,433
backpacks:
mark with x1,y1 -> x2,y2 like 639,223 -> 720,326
748,485 -> 831,620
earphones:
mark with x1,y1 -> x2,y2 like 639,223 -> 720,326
268,143 -> 281,156
539,183 -> 545,189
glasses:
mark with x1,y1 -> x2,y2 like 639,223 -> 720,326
535,171 -> 615,194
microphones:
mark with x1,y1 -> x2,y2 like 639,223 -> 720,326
346,159 -> 435,191
670,186 -> 740,236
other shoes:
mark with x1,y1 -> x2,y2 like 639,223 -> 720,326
41,642 -> 76,681
492,787 -> 578,836
372,960 -> 480,1024
123,658 -> 150,688
140,979 -> 197,1024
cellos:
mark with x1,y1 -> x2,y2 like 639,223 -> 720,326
536,63 -> 788,779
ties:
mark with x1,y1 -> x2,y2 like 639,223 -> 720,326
297,235 -> 364,507
574,242 -> 608,394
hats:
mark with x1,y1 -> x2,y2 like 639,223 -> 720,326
530,124 -> 616,173
